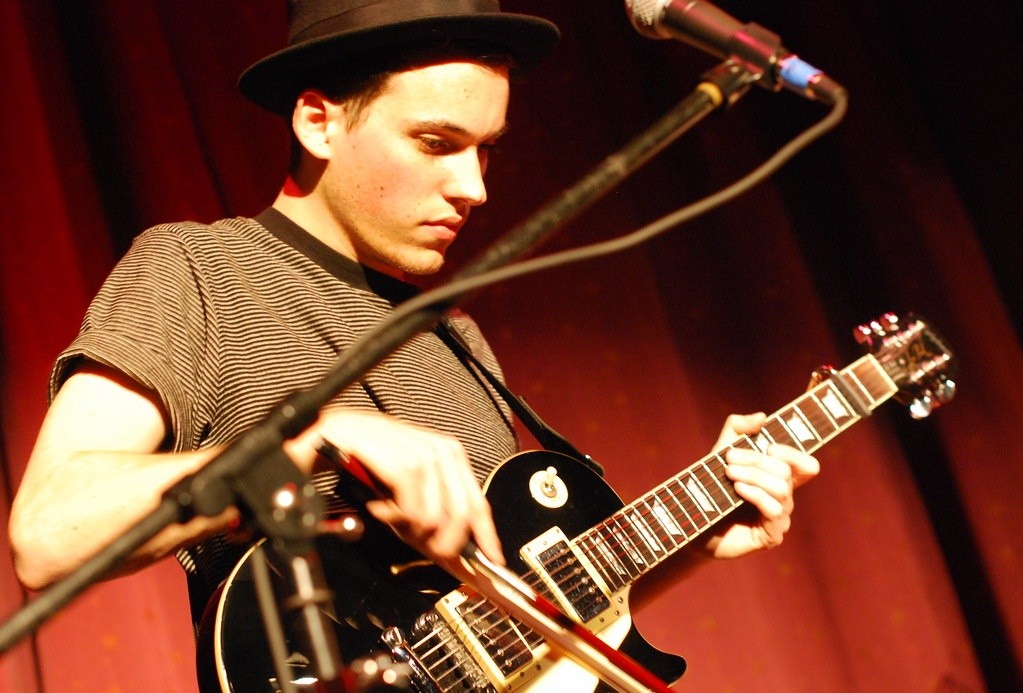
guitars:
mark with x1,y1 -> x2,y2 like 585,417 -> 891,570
194,310 -> 961,693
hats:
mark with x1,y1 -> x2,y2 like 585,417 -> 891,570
235,0 -> 563,113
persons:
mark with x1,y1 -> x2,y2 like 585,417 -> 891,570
7,1 -> 822,693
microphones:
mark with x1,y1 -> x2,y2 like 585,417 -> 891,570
625,0 -> 845,102
